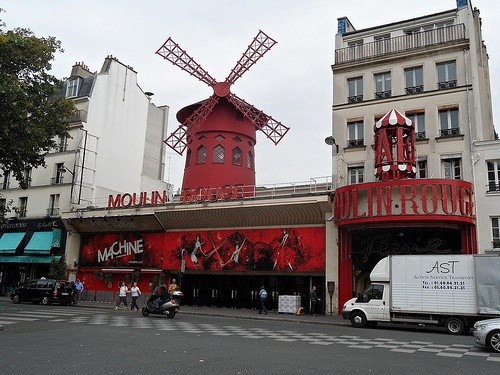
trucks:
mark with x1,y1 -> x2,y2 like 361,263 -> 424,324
341,251 -> 500,335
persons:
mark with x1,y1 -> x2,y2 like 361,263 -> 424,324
169,279 -> 180,310
258,286 -> 268,315
75,279 -> 83,305
114,281 -> 130,311
156,284 -> 172,309
126,281 -> 141,312
310,286 -> 321,316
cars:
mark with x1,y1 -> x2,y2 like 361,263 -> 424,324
472,316 -> 500,351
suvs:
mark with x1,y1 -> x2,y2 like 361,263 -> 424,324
9,279 -> 76,305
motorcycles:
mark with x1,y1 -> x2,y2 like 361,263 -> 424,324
141,289 -> 183,319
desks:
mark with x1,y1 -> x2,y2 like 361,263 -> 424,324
278,295 -> 301,314
